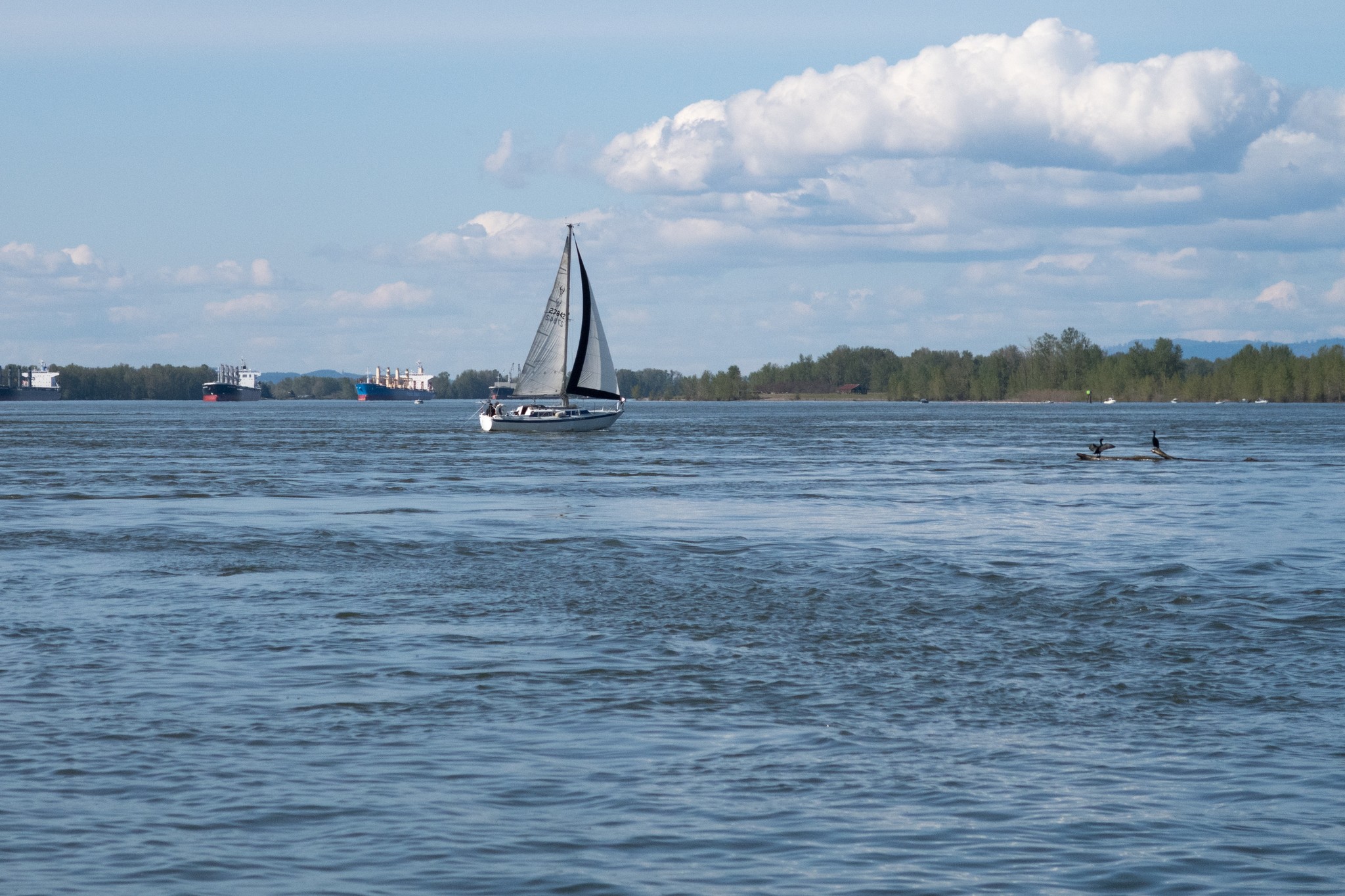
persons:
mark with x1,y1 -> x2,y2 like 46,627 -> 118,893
493,401 -> 499,408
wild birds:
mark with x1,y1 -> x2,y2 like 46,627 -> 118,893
1089,439 -> 1116,455
1152,430 -> 1159,448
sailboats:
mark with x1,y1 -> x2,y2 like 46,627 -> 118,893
478,223 -> 627,431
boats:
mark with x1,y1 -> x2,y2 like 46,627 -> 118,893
920,398 -> 929,403
1104,394 -> 1117,404
355,360 -> 436,401
1238,399 -> 1252,403
414,398 -> 423,404
0,359 -> 62,401
1215,400 -> 1225,404
1255,397 -> 1269,404
1171,398 -> 1178,404
1042,400 -> 1054,403
488,374 -> 560,400
202,356 -> 261,402
